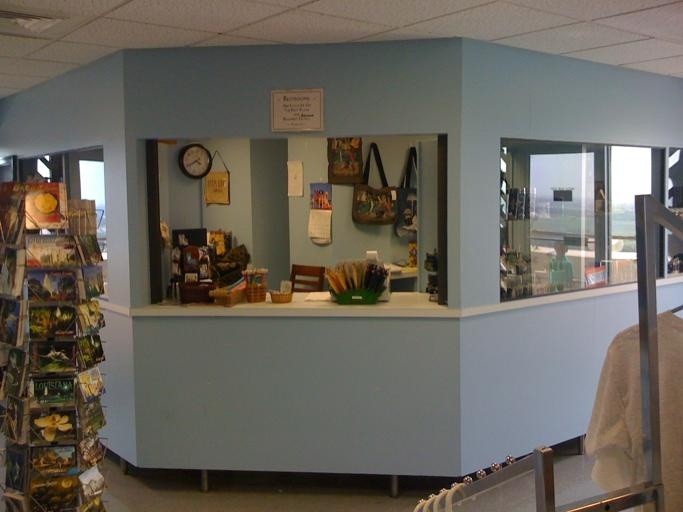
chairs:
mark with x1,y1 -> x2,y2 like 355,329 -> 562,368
289,264 -> 324,294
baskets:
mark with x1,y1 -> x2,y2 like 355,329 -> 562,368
270,292 -> 293,304
245,285 -> 267,302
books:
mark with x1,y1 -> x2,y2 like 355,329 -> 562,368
309,182 -> 333,245
0,181 -> 107,512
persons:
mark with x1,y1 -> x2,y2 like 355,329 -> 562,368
548,240 -> 574,292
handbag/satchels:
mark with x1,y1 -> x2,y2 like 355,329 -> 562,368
203,151 -> 231,205
352,142 -> 420,237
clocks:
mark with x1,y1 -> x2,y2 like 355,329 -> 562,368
180,142 -> 211,178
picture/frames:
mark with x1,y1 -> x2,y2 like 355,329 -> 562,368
270,87 -> 325,134
